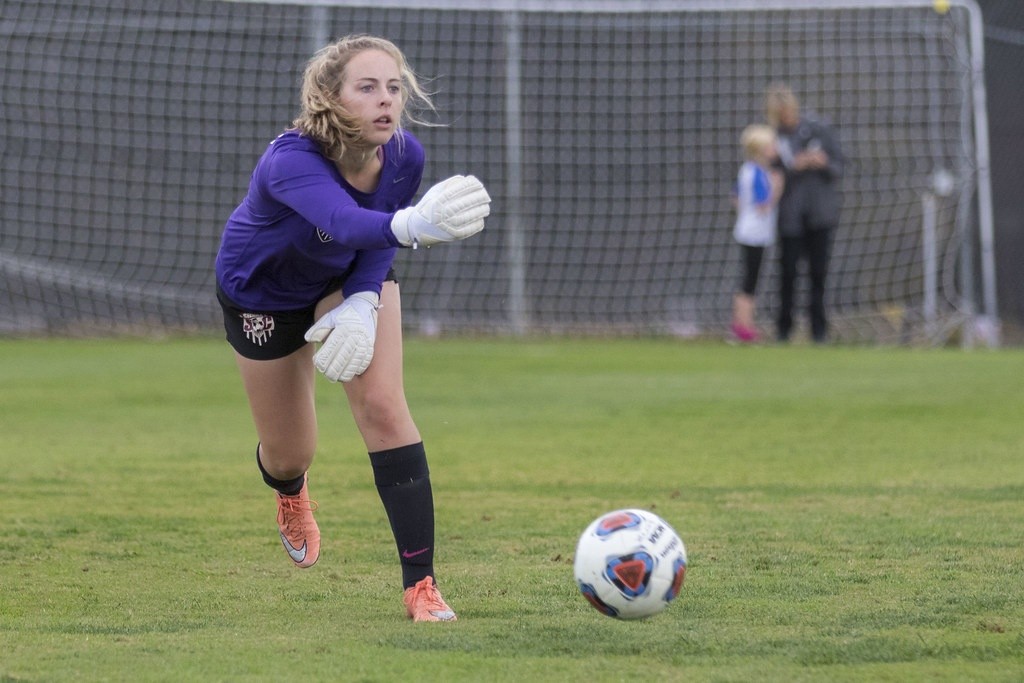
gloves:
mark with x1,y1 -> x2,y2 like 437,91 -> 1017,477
304,291 -> 384,383
391,175 -> 491,250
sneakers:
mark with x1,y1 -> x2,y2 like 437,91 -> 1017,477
403,576 -> 457,622
273,470 -> 321,569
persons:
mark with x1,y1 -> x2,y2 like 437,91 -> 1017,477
215,37 -> 491,622
767,88 -> 845,344
730,124 -> 785,344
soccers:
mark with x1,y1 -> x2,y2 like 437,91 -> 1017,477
573,507 -> 689,620
